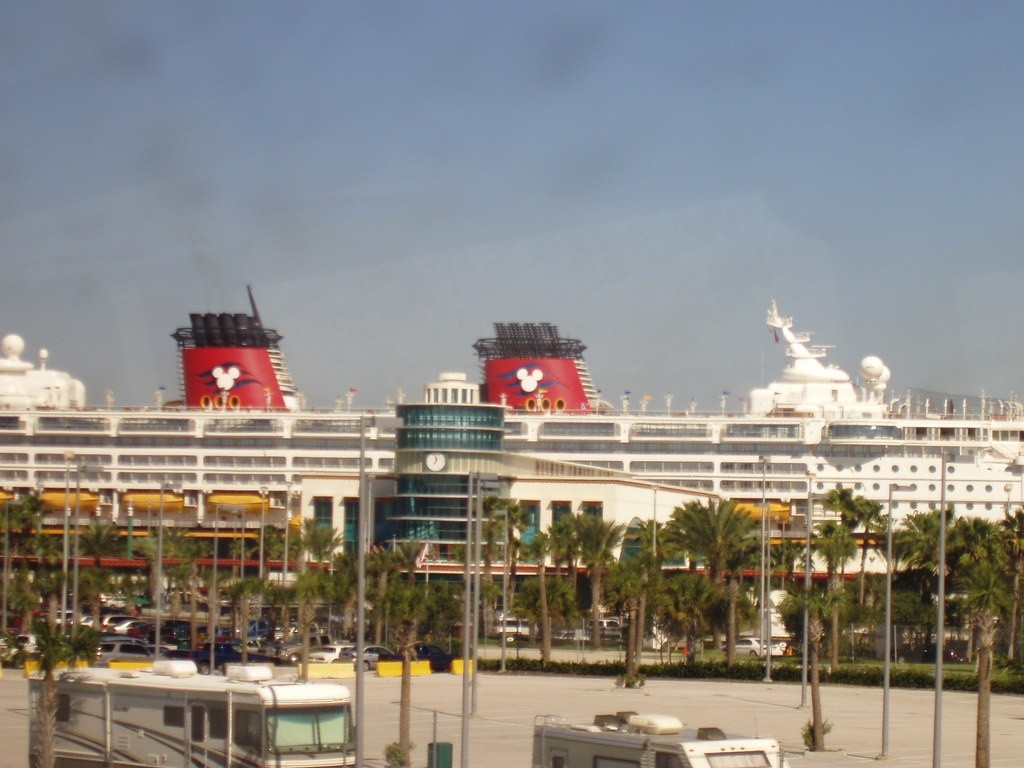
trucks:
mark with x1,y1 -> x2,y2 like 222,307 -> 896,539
530,710 -> 789,767
24,657 -> 356,767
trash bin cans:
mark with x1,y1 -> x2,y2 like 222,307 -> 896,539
427,742 -> 454,768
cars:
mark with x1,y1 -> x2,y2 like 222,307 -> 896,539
0,585 -> 544,669
720,637 -> 783,657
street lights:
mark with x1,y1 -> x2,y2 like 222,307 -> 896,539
154,480 -> 182,662
758,454 -> 771,661
755,501 -> 774,682
932,451 -> 977,768
800,490 -> 829,707
353,414 -> 404,768
879,483 -> 915,757
209,502 -> 243,678
459,469 -> 500,768
470,480 -> 503,716
74,465 -> 105,658
2,500 -> 28,637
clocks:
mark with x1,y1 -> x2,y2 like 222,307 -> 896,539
423,453 -> 449,474
